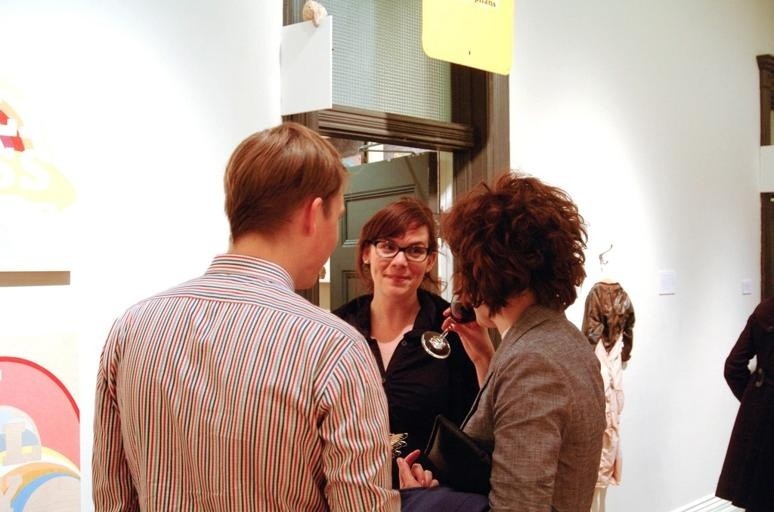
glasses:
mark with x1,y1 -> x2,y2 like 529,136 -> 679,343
365,239 -> 432,263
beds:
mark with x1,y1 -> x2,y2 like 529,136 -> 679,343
0,356 -> 79,512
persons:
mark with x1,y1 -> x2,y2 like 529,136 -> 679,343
389,171 -> 606,512
328,194 -> 481,483
712,296 -> 773,511
87,123 -> 400,510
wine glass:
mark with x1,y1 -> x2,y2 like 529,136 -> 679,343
422,293 -> 479,359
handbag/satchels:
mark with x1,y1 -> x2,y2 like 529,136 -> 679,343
419,413 -> 494,498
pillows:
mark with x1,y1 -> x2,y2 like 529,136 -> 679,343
0,406 -> 41,451
